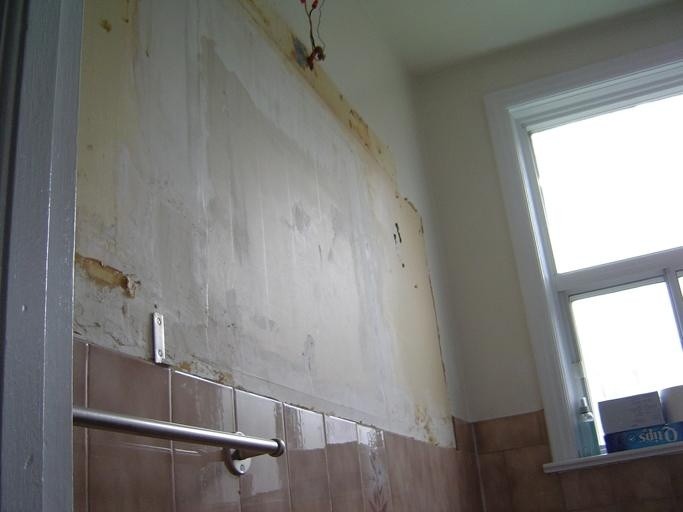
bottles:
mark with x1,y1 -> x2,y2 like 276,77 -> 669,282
576,405 -> 599,458
